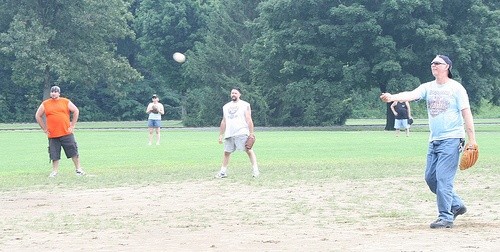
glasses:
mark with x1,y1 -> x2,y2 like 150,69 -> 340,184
431,61 -> 444,65
153,97 -> 156,99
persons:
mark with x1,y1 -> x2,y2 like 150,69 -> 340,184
146,95 -> 164,144
36,86 -> 83,176
390,100 -> 413,137
379,54 -> 477,227
219,87 -> 258,177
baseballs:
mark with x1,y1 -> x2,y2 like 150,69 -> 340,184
173,52 -> 185,63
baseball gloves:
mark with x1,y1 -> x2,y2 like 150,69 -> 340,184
407,118 -> 413,125
245,134 -> 255,150
151,105 -> 158,114
460,143 -> 479,170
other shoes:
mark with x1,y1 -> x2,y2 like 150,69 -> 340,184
76,169 -> 88,176
48,169 -> 58,178
214,172 -> 227,178
429,217 -> 453,229
454,205 -> 467,219
251,169 -> 259,177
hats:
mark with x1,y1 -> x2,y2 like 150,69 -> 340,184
439,54 -> 453,78
152,93 -> 157,98
